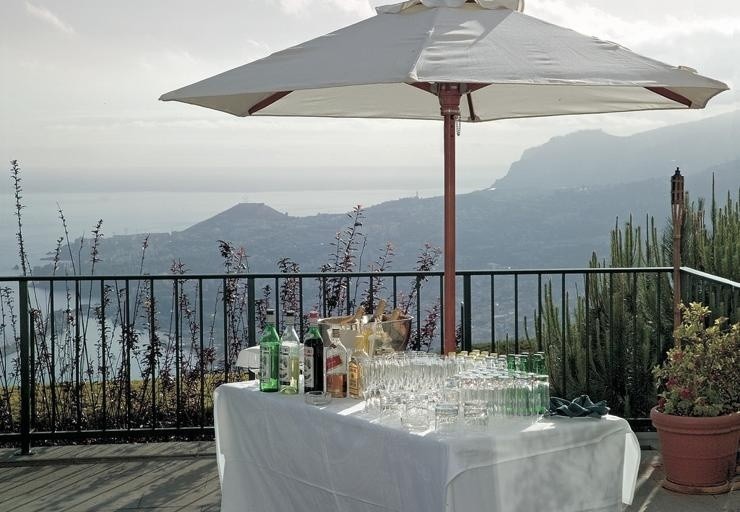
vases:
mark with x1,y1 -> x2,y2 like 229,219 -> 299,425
647,405 -> 740,496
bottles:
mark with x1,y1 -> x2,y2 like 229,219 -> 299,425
304,311 -> 323,396
325,327 -> 349,399
259,308 -> 280,394
348,336 -> 368,399
337,299 -> 405,323
278,309 -> 299,396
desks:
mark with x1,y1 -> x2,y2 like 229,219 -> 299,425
238,342 -> 305,380
217,378 -> 629,512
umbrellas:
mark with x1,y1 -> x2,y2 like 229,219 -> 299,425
158,1 -> 731,357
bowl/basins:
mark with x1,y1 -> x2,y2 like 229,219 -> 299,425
319,312 -> 414,351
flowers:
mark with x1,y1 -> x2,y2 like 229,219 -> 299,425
650,301 -> 740,416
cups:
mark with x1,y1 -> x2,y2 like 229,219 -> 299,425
368,349 -> 551,431
